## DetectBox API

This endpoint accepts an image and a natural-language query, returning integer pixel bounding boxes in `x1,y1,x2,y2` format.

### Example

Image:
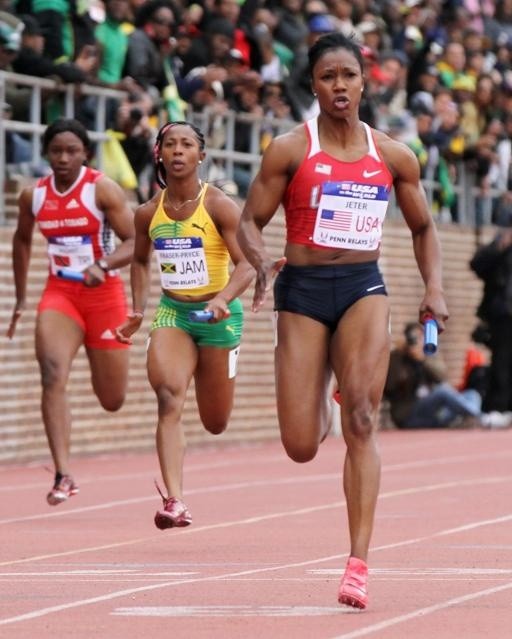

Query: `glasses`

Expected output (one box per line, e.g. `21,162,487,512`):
154,15,174,30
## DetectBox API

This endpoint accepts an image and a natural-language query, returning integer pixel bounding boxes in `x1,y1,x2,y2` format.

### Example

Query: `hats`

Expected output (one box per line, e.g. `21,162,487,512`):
307,14,332,30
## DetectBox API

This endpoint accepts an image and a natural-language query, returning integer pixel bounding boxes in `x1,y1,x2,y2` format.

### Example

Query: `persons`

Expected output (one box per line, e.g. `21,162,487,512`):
234,30,450,609
1,1,512,228
5,117,135,507
382,322,512,429
114,120,258,531
468,191,512,413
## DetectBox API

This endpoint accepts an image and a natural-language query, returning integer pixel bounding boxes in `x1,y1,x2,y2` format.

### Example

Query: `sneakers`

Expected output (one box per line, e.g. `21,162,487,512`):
152,480,194,530
481,409,512,428
336,556,369,610
46,470,80,507
